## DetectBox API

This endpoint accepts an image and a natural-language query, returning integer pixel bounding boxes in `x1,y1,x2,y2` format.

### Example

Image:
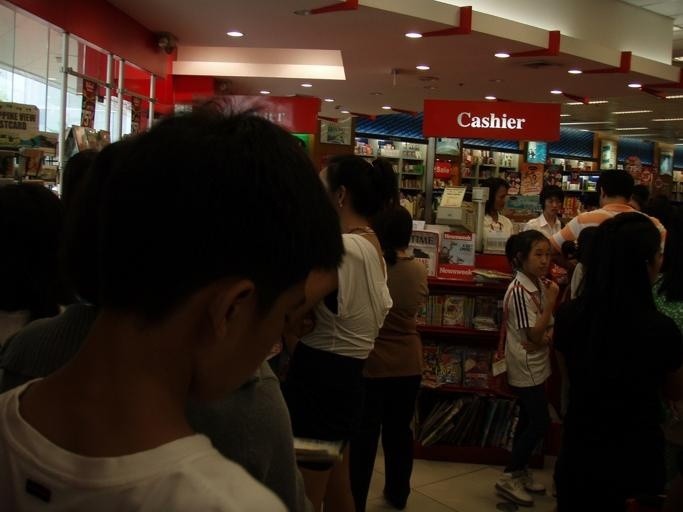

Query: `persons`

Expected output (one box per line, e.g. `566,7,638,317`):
481,176,565,243
0,96,347,512
549,170,683,511
495,230,559,507
271,155,428,511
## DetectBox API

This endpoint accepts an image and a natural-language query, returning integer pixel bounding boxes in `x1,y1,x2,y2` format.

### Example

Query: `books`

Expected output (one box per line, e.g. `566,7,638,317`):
411,227,520,453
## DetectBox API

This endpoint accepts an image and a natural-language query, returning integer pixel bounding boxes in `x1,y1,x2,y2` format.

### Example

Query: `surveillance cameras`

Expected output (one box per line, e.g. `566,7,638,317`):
159,33,175,54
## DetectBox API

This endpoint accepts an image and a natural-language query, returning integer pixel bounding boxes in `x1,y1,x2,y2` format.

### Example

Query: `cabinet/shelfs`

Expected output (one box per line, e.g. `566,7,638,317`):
542,151,600,223
354,135,427,222
404,276,547,470
671,169,682,205
432,135,522,223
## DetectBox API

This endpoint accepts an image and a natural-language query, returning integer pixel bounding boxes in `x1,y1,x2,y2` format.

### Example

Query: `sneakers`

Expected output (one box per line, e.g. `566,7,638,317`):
494,470,546,507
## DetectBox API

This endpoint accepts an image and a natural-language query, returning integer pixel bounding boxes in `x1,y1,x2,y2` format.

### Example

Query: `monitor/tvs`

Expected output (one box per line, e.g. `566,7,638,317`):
436,201,479,233
